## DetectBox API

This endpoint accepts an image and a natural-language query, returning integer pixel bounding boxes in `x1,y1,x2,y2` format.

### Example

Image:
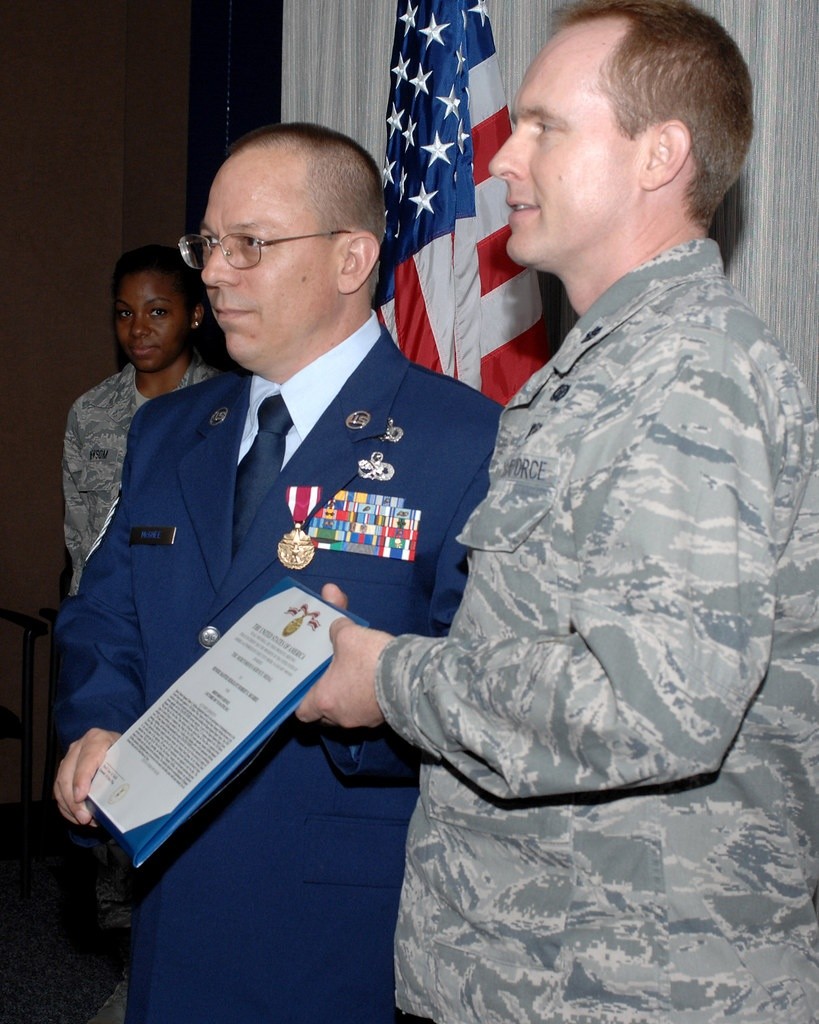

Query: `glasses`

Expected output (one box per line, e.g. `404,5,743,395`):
178,229,354,270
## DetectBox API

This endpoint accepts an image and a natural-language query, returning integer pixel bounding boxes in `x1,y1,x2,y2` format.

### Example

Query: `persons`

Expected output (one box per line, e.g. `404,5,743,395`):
61,245,219,597
291,66,817,1024
53,121,505,1023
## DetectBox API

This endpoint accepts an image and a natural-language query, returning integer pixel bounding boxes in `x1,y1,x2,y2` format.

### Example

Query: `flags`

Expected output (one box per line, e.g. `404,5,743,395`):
376,0,548,409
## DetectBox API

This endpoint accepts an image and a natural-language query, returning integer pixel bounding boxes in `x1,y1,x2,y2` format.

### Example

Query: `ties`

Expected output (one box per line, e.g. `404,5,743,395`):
231,394,293,565
